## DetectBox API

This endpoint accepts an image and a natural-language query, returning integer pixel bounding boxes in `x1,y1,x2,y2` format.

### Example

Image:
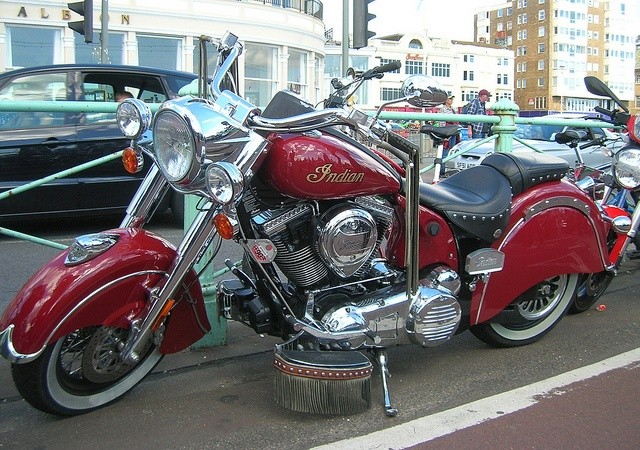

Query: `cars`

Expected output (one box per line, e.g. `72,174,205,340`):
444,113,627,177
384,123,409,139
1,64,210,231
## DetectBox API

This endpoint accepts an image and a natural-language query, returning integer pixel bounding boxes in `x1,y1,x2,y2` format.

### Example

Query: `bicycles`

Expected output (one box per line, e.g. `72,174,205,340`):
554,131,614,195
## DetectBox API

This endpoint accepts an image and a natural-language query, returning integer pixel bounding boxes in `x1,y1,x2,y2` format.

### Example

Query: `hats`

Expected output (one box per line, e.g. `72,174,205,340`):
479,90,492,96
448,94,455,98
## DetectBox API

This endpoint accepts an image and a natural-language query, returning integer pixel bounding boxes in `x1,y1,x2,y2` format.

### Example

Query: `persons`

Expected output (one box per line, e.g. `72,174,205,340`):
467,90,492,138
439,95,458,158
115,91,134,103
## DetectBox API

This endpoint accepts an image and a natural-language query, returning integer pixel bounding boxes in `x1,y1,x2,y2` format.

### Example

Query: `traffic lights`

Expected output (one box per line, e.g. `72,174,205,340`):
354,0,376,49
67,1,93,42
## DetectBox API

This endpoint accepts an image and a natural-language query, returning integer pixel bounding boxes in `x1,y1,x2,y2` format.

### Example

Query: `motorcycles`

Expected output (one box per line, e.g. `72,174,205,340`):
570,77,639,315
0,29,613,417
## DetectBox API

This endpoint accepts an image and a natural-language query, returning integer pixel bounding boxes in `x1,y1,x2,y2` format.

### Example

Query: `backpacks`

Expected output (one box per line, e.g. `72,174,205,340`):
459,99,479,128
